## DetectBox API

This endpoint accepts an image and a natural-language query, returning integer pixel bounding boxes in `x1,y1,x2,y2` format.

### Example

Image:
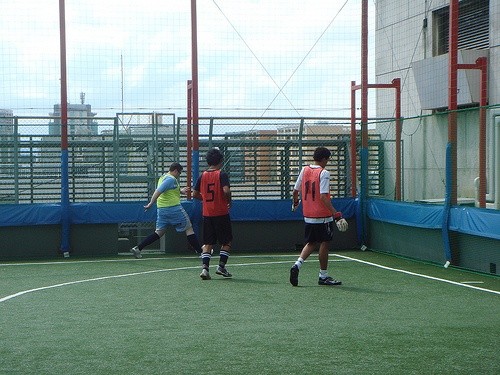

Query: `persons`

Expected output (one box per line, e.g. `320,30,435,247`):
192,149,234,280
289,147,349,287
131,162,214,259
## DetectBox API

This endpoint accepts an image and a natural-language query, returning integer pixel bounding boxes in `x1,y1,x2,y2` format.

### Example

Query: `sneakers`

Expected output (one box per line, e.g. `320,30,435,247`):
318,277,341,285
200,269,211,280
197,249,214,256
290,265,299,287
132,246,142,258
215,266,232,277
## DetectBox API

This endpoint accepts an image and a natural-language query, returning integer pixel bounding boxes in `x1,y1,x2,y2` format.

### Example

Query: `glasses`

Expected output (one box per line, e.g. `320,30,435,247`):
177,169,181,174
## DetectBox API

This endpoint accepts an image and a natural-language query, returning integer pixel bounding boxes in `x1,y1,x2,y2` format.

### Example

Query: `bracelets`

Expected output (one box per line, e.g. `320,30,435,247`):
333,212,342,218
294,199,299,204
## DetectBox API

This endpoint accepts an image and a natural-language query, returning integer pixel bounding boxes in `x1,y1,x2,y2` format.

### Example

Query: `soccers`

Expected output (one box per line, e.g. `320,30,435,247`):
202,244,213,256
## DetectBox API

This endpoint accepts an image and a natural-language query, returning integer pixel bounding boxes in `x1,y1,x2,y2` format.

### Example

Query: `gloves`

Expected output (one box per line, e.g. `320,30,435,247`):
334,212,348,232
292,200,301,212
